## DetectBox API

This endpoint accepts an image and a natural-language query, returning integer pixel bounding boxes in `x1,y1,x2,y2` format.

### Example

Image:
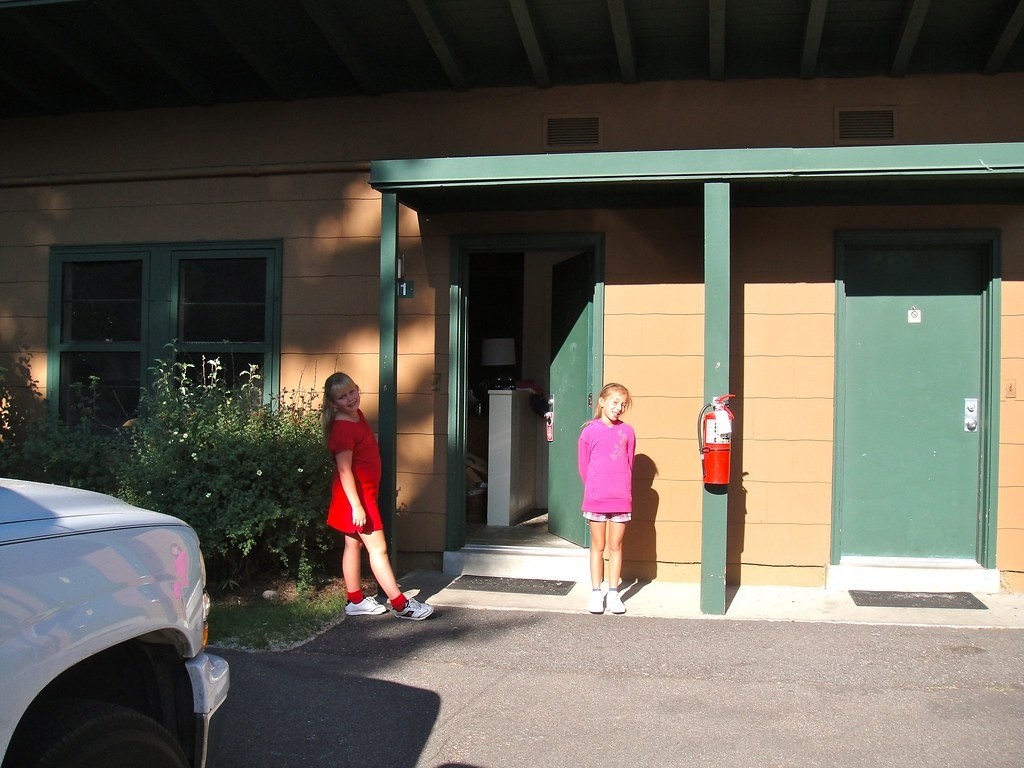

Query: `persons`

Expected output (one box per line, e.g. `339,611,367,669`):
578,382,637,614
319,371,436,621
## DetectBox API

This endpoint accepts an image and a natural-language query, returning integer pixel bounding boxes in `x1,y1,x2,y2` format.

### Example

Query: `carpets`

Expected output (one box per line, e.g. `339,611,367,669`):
846,590,989,606
445,574,578,596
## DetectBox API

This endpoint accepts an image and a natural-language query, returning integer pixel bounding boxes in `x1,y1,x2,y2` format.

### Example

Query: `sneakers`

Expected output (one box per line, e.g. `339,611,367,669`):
389,597,435,620
603,593,626,614
344,595,387,615
588,591,604,613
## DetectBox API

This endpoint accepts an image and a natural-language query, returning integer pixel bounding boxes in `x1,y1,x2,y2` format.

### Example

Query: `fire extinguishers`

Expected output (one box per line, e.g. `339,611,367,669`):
698,393,736,484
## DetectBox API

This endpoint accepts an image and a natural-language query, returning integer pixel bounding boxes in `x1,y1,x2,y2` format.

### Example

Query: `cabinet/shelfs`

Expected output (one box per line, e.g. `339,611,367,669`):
489,390,550,527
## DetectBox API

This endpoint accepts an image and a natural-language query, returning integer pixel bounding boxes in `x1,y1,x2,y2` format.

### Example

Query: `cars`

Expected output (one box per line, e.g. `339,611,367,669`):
0,476,232,767
